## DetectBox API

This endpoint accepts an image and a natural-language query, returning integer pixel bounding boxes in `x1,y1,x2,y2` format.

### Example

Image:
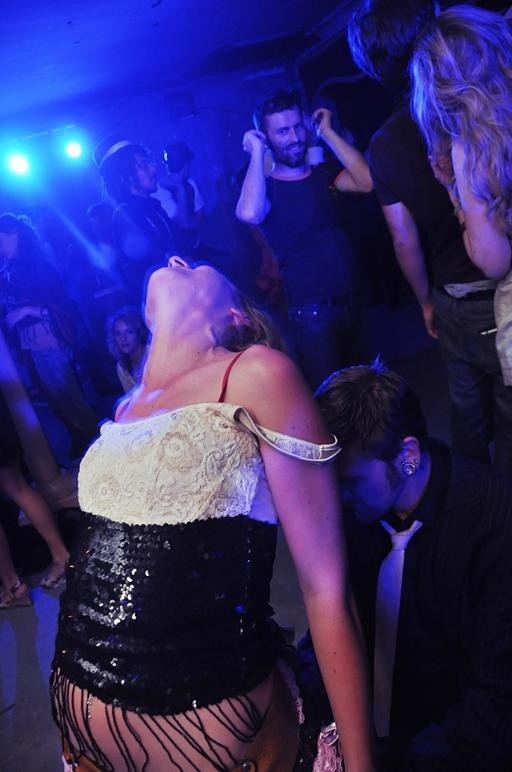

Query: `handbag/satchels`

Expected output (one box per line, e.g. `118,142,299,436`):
54,296,93,351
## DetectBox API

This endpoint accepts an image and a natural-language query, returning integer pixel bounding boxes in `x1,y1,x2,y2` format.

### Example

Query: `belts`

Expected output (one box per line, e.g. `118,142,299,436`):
371,520,423,738
442,287,494,302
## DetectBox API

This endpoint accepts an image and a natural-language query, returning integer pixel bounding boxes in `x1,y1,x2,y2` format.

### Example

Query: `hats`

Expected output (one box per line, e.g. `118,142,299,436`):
160,141,196,164
93,134,142,175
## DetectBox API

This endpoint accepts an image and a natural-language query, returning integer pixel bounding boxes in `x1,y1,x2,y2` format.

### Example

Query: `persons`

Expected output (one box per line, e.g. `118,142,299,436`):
1,2,511,771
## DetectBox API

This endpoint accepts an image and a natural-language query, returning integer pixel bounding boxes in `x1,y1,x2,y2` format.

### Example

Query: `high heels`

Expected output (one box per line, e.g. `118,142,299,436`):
0,577,33,609
38,552,72,588
